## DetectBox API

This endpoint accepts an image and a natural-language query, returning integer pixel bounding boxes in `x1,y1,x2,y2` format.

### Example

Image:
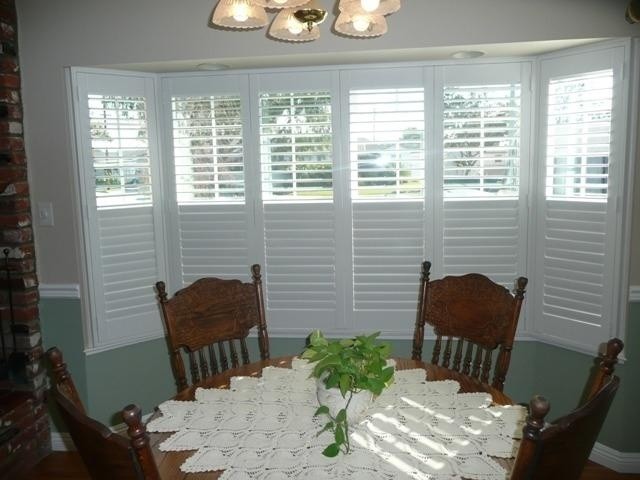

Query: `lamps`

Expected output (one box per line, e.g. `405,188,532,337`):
206,0,403,46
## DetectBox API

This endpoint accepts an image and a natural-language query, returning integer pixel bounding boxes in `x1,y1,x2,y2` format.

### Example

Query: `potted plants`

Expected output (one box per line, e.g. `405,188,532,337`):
297,325,397,460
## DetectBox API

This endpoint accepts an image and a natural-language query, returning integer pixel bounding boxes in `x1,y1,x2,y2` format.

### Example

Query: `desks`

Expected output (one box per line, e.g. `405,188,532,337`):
135,350,529,479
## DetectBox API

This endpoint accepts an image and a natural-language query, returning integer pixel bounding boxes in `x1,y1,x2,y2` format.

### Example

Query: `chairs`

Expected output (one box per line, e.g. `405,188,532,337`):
42,343,166,480
409,258,529,396
155,262,273,393
505,337,625,480
